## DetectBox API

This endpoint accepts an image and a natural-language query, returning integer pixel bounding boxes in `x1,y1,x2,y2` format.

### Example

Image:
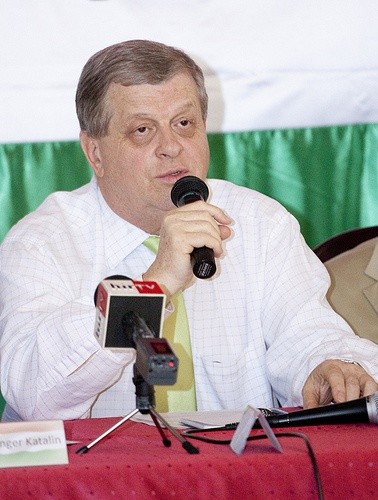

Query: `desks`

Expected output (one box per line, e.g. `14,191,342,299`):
0,416,378,500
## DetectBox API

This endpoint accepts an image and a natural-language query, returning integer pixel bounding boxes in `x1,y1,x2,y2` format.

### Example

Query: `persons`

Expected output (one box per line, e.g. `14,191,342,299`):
0,40,378,420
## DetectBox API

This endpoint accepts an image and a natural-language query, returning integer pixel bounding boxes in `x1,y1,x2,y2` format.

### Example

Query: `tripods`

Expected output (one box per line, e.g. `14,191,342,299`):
75,365,200,457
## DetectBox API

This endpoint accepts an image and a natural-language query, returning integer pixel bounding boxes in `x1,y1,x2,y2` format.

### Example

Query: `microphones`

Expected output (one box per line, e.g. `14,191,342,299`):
171,175,217,279
94,275,179,387
225,393,378,431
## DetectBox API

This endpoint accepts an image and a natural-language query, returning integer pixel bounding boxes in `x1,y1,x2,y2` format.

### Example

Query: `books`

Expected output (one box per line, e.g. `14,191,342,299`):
179,408,282,430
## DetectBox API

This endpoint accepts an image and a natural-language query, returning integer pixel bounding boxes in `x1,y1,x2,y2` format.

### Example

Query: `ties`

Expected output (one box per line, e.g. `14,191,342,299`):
144,237,196,413
